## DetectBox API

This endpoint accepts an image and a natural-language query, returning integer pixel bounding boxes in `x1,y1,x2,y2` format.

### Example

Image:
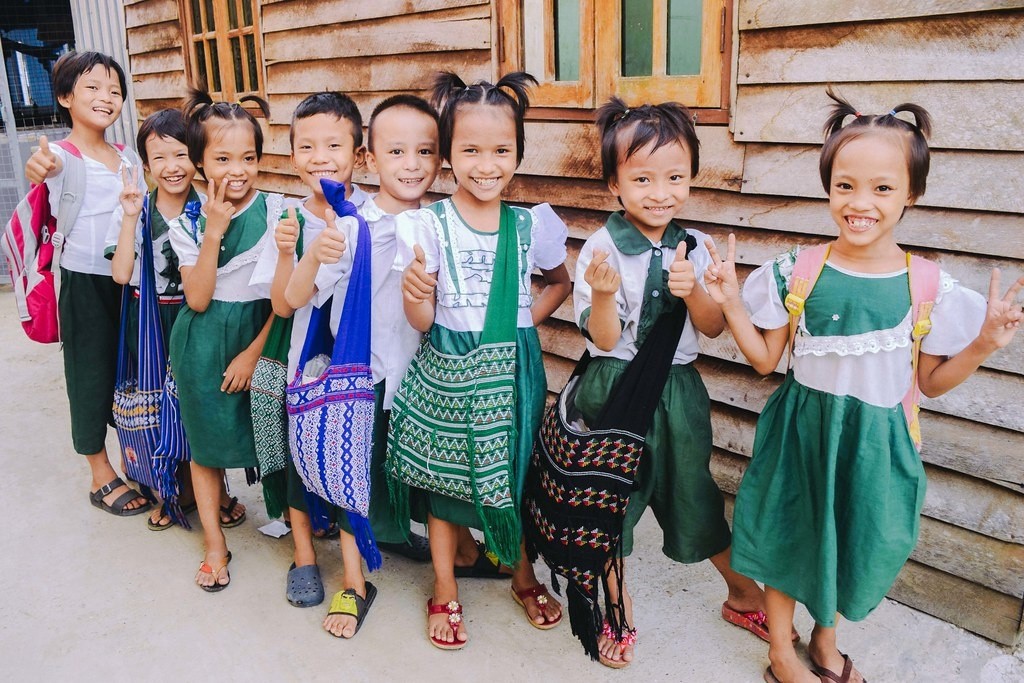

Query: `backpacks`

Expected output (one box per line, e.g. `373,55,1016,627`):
111,380,165,491
785,244,941,454
0,139,140,345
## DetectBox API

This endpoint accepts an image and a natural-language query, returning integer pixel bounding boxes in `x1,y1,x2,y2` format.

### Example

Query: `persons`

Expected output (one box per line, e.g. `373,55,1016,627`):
573,94,801,668
26,51,153,517
104,70,572,652
704,80,1023,683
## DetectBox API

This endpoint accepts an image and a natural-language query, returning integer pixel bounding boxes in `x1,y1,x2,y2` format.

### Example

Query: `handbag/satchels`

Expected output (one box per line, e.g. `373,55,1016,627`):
383,329,523,573
285,362,383,574
249,355,284,482
520,375,646,663
152,357,193,532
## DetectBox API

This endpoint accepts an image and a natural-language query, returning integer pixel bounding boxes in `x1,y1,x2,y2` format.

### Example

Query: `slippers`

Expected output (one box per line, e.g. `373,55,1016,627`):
199,550,232,592
452,539,515,580
763,665,830,683
596,607,638,669
325,581,378,639
722,599,801,647
286,561,325,608
425,597,468,650
219,496,246,528
510,582,563,630
374,530,434,563
147,500,197,531
89,473,151,516
809,646,867,683
285,515,340,539
